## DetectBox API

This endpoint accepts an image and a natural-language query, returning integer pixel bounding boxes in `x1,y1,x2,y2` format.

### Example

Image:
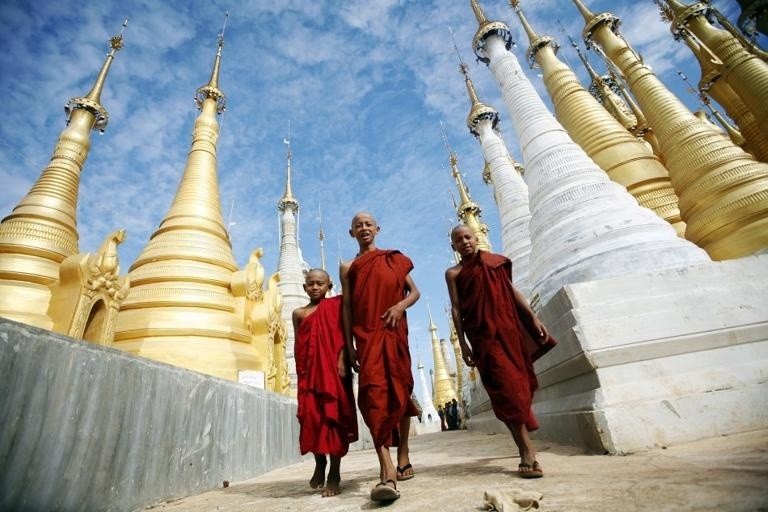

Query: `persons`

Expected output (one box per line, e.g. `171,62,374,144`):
444,225,556,478
437,399,458,432
340,213,421,501
292,269,348,498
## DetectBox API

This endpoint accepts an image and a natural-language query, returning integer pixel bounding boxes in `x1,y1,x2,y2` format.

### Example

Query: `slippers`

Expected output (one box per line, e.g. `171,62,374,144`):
397,463,415,480
518,460,543,477
371,479,400,501
